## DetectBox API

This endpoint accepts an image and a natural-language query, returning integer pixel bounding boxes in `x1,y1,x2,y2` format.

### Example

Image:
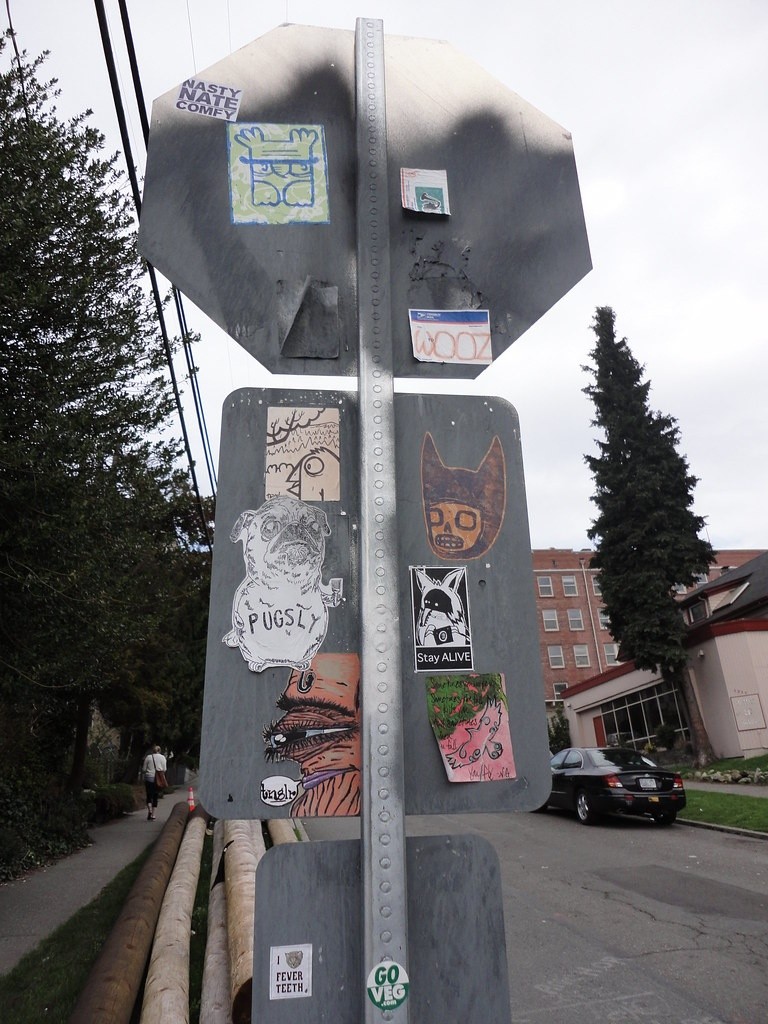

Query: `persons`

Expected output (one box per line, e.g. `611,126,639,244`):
143,746,166,820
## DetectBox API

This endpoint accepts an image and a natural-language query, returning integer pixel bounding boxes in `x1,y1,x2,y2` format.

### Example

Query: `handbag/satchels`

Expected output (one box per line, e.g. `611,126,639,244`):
154,770,168,790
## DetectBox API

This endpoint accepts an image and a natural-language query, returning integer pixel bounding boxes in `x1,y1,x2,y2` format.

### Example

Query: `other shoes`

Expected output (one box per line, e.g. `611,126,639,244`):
147,811,153,820
151,816,155,821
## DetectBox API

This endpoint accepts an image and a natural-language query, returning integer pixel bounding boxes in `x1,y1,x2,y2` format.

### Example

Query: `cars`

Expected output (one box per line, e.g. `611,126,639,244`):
548,747,687,827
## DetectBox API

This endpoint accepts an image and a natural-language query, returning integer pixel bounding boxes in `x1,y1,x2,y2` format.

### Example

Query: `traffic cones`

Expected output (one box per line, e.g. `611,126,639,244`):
187,786,195,811
